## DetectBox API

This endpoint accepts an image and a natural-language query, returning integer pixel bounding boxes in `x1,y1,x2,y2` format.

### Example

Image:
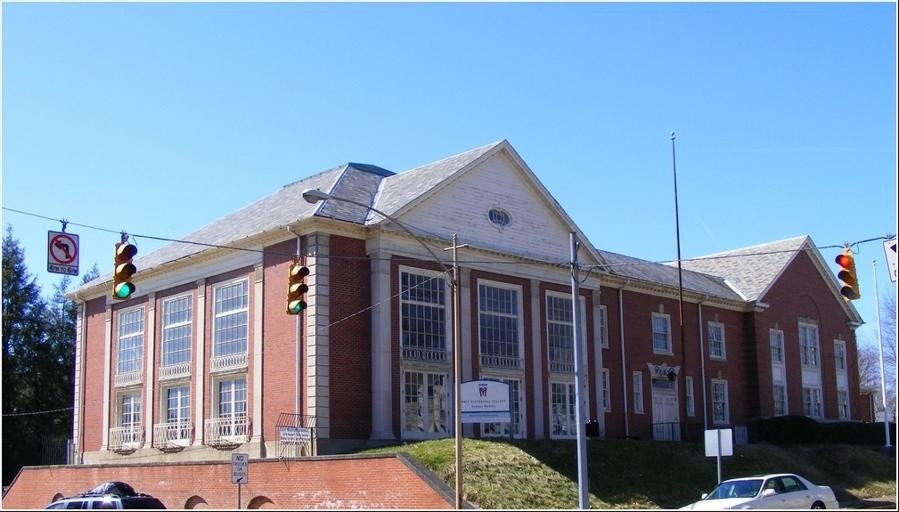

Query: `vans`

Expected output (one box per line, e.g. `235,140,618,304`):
44,493,168,509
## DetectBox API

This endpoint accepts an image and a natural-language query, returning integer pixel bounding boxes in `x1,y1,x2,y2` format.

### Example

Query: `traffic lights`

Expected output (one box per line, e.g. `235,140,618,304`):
835,247,862,302
112,243,138,301
285,264,310,316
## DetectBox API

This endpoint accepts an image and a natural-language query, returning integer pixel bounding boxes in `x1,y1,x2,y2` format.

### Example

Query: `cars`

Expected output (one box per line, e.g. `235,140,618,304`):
676,472,840,511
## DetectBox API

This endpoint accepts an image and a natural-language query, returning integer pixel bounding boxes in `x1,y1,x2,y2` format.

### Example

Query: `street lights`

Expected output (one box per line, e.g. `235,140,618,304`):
301,187,469,509
